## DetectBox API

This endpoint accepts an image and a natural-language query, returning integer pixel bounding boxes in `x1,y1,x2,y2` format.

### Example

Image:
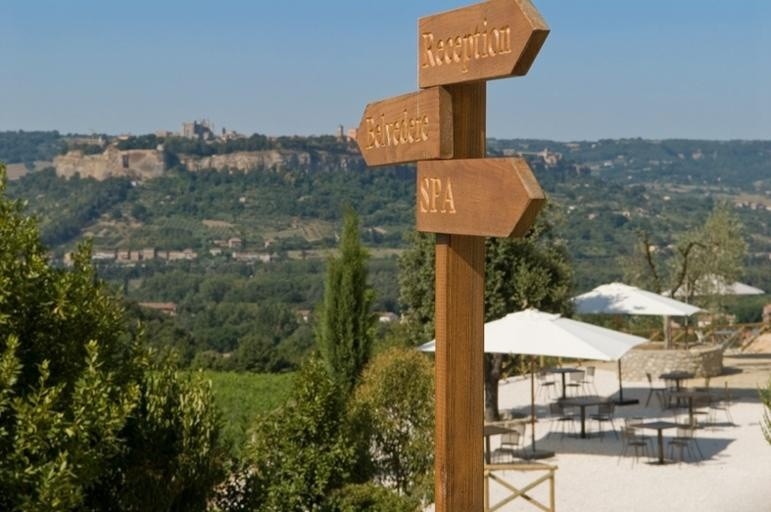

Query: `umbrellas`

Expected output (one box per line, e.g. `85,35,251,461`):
416,304,650,451
557,278,708,401
655,269,765,340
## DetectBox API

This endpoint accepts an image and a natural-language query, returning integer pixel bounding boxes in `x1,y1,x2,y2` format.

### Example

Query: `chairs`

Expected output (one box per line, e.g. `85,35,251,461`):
493,366,733,468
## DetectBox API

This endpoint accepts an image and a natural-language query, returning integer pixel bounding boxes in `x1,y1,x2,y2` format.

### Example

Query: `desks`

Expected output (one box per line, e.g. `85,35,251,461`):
484,425,516,464
